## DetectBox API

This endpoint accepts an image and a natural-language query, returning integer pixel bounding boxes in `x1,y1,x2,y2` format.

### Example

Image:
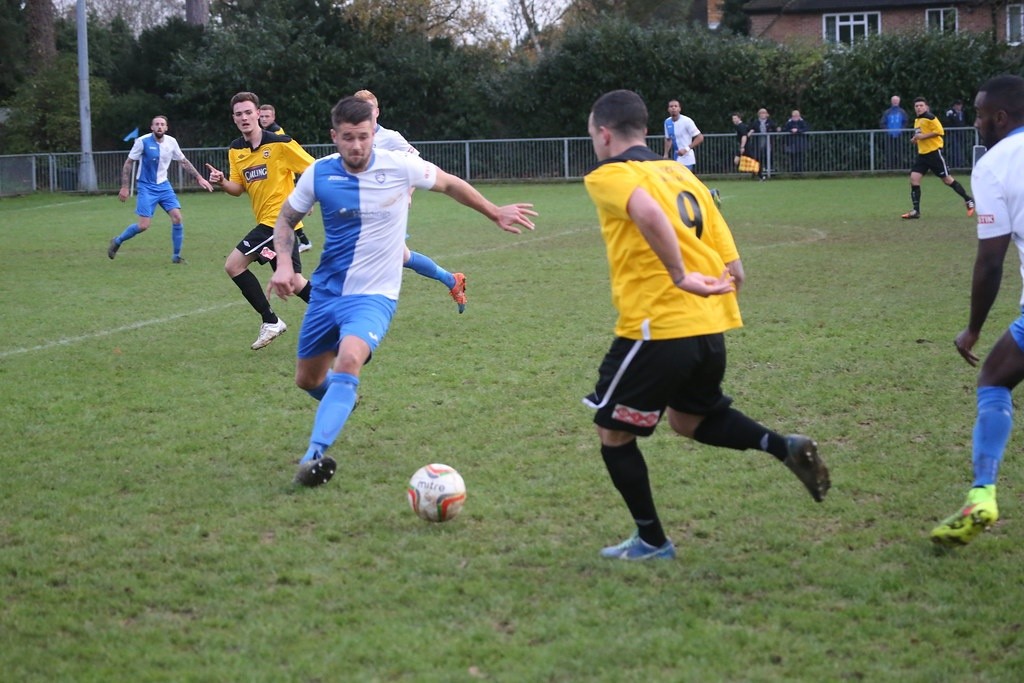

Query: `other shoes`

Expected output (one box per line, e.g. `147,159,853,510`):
712,189,721,209
901,209,920,218
173,256,188,264
966,197,975,217
108,235,120,260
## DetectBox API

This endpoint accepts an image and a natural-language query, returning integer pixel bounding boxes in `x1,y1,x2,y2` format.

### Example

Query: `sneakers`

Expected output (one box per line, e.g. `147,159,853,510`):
930,486,998,549
780,434,831,504
601,530,676,562
294,456,336,488
449,273,467,314
250,317,286,351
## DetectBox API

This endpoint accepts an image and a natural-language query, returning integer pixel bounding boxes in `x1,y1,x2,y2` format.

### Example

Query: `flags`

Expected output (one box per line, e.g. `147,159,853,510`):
124,128,138,142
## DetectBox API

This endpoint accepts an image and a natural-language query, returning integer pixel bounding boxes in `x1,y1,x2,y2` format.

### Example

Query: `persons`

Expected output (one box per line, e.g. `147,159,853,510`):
108,115,214,265
206,91,316,350
663,95,973,218
353,89,466,313
266,97,539,493
931,73,1024,547
583,89,832,560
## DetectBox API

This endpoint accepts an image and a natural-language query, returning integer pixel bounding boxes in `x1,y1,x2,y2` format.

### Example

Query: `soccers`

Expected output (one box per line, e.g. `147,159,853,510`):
407,462,468,523
